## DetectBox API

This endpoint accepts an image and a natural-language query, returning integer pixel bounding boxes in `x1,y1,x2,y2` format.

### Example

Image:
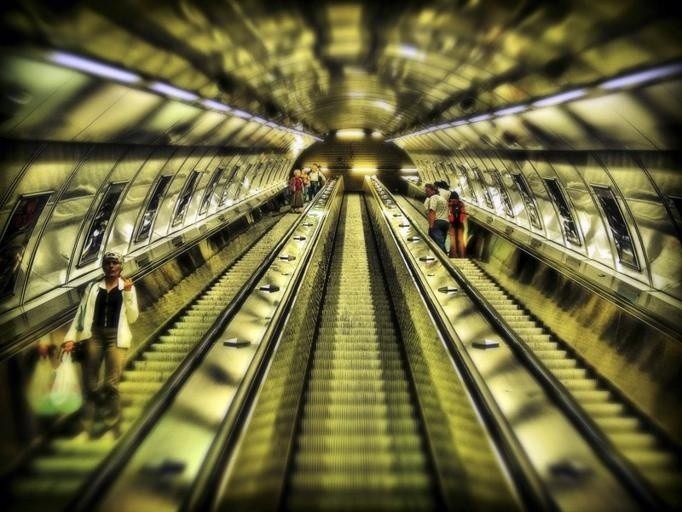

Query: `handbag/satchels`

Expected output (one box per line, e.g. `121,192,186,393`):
290,184,296,191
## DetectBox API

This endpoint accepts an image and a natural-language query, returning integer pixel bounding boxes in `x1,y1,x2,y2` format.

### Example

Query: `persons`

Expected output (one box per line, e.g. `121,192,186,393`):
289,163,326,215
59,252,141,435
423,179,469,260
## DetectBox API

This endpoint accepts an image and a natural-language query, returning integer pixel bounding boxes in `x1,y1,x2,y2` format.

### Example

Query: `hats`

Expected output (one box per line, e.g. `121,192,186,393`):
101,252,124,271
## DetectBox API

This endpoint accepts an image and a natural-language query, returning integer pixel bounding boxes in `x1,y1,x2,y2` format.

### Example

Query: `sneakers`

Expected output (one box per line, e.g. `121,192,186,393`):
290,209,298,213
91,406,122,436
